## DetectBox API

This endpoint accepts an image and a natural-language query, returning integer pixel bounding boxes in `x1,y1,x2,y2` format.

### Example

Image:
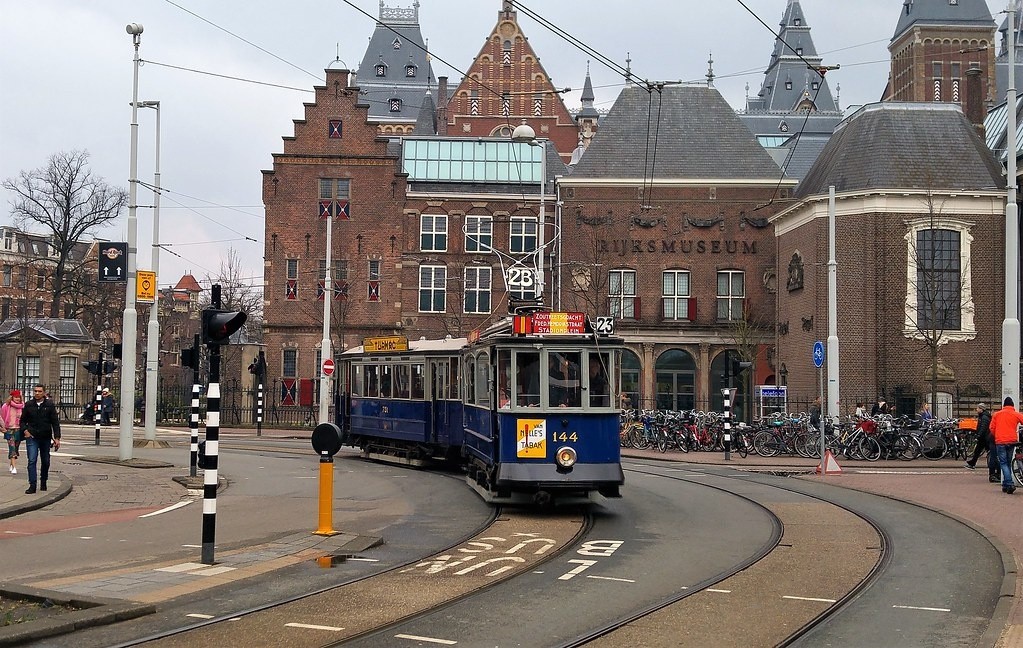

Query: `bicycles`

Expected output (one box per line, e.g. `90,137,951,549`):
1012,428,1023,486
620,400,991,461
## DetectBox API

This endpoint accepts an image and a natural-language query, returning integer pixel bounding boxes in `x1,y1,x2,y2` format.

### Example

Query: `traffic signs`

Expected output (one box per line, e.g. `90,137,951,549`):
97,242,127,283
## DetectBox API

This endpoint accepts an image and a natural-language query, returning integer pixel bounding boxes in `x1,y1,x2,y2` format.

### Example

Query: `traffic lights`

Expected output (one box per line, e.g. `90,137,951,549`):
82,361,97,375
200,308,247,347
181,347,195,369
247,357,260,374
104,361,117,374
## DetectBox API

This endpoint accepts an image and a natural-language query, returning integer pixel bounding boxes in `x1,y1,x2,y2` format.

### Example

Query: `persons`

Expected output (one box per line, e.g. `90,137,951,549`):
855,396,896,418
102,387,113,425
964,397,1023,494
621,392,634,412
527,354,608,408
18,384,61,493
810,396,822,429
0,388,25,474
919,402,932,420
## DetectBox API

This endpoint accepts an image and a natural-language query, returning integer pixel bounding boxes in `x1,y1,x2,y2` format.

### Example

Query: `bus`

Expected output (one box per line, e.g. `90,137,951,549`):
334,224,626,509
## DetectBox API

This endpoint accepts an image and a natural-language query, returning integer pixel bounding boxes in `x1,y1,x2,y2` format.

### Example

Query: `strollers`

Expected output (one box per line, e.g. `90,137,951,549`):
78,398,97,425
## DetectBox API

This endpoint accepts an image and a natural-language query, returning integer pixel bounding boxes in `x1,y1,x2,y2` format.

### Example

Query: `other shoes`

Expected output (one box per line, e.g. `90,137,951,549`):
101,422,113,426
1002,485,1017,494
965,460,976,469
25,486,47,494
988,473,1001,483
8,464,18,474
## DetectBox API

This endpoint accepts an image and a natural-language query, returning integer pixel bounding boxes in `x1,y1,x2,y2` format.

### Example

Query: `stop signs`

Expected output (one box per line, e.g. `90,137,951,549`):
323,359,334,375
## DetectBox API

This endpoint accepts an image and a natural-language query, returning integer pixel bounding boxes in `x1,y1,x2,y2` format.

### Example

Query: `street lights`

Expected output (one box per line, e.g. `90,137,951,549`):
117,20,145,461
721,348,753,458
127,101,162,442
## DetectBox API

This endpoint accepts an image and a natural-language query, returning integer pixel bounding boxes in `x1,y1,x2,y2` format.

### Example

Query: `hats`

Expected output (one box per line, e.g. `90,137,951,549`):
102,387,110,393
978,402,987,410
1003,397,1015,407
9,390,22,397
877,400,886,409
